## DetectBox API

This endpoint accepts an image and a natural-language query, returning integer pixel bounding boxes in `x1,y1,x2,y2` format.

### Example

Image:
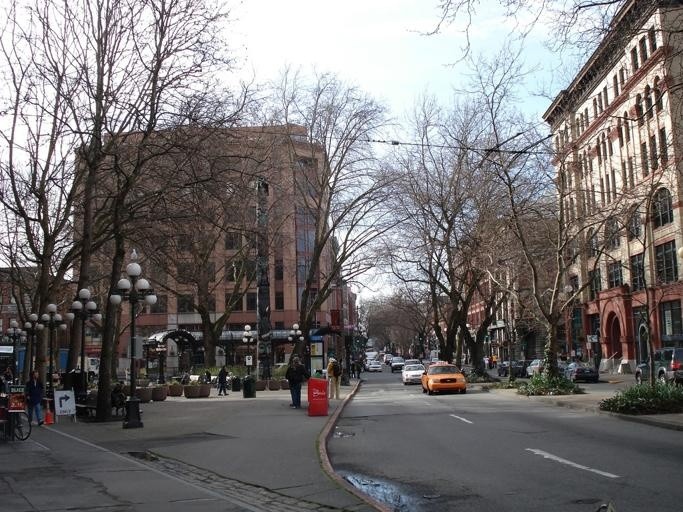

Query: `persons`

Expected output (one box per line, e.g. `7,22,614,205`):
325,356,341,400
492,354,498,368
285,357,308,408
216,365,229,397
24,369,44,427
350,359,364,379
487,355,493,369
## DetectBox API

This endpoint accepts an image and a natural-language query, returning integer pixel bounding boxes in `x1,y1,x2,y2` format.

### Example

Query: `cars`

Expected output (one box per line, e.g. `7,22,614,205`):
365,348,467,395
498,359,599,383
275,363,288,369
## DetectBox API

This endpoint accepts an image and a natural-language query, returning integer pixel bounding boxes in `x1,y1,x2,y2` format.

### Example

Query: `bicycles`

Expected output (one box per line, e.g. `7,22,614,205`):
7,412,32,442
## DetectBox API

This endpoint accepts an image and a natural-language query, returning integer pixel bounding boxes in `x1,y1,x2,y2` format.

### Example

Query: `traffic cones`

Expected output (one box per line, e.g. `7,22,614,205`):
44,400,56,426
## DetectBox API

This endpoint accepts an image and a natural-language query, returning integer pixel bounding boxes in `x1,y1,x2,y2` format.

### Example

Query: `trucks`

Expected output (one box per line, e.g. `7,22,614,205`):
76,355,100,380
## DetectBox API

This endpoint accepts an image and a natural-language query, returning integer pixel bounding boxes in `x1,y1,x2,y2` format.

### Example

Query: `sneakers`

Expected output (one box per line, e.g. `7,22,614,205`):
37,420,45,427
218,394,229,396
289,404,300,409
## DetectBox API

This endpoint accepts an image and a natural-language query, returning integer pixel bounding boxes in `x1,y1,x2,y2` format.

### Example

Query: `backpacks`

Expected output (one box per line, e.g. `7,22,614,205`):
331,360,344,377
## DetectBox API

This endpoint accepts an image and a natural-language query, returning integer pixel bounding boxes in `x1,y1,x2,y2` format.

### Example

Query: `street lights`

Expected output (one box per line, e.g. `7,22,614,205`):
24,312,44,373
564,285,578,361
65,286,102,404
241,324,255,374
5,320,27,385
464,322,470,364
110,259,158,431
36,303,66,399
287,323,305,355
153,344,166,384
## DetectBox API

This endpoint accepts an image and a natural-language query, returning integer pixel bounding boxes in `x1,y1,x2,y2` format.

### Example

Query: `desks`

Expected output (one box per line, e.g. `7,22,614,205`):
171,376,182,383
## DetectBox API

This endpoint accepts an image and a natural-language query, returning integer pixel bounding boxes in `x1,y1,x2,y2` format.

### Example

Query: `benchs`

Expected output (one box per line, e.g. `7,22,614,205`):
76,403,126,422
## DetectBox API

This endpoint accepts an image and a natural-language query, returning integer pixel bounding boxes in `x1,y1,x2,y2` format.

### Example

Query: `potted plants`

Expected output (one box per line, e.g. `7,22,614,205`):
136,379,152,403
268,376,280,390
197,379,211,397
183,380,200,397
153,383,167,402
231,376,240,391
167,378,183,395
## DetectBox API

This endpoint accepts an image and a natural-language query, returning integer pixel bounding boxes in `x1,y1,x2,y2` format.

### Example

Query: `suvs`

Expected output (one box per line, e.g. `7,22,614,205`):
634,347,683,386
